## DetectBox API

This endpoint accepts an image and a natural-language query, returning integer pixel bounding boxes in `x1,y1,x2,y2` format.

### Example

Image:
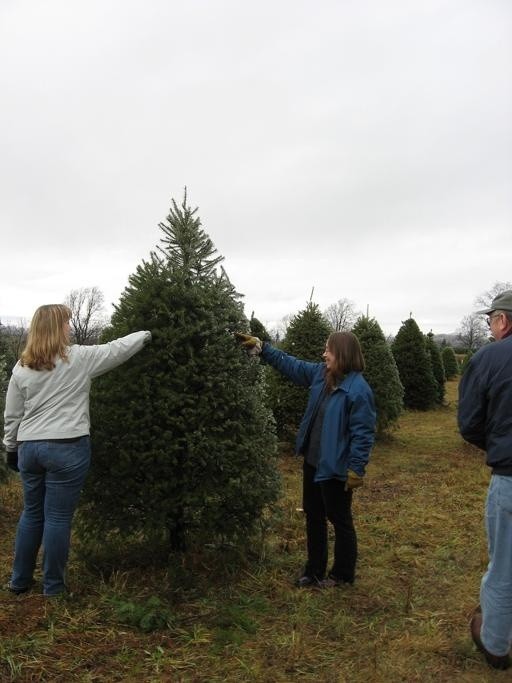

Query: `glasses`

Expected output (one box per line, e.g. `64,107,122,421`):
486,315,499,327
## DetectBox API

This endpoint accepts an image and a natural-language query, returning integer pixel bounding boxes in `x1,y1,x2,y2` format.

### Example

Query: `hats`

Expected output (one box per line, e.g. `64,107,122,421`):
476,290,512,314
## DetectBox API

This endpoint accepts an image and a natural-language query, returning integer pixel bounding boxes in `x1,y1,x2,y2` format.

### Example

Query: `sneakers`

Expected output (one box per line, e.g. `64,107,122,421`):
472,615,510,669
9,578,36,593
296,575,314,586
318,578,338,588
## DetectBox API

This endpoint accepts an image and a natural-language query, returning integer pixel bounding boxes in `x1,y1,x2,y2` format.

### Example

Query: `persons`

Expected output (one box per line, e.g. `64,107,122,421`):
236,330,377,593
2,303,153,599
455,287,511,670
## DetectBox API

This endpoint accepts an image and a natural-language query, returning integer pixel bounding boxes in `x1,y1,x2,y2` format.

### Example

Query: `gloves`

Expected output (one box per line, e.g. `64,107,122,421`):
6,451,19,472
344,469,362,492
234,331,264,352
